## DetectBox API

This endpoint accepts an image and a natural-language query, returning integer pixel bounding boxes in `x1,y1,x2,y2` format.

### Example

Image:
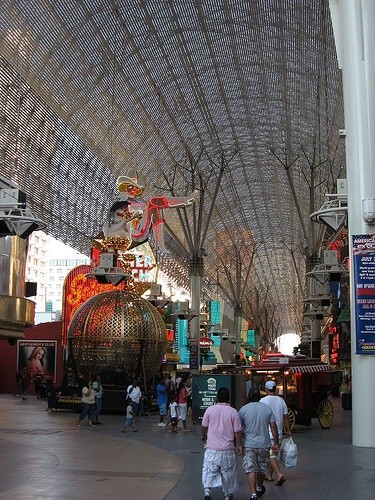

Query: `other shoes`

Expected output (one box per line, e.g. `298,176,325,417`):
204,489,212,500
167,426,182,434
182,428,193,433
90,421,101,426
222,493,233,500
77,420,81,427
122,426,139,433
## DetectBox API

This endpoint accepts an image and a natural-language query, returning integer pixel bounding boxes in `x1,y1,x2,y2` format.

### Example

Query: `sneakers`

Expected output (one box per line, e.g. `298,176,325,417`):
250,494,259,500
257,485,266,497
157,422,166,427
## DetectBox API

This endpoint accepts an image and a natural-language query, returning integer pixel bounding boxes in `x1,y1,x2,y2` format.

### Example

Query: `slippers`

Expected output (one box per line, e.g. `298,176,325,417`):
264,475,275,481
275,479,286,486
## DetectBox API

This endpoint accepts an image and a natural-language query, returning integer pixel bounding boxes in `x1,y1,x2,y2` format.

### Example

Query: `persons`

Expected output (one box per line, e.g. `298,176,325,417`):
34,370,53,400
176,380,192,432
186,376,192,415
126,379,147,427
157,379,167,427
121,400,138,432
10,367,29,399
76,379,97,427
25,347,46,376
235,388,280,500
91,374,103,425
167,391,182,432
45,376,57,412
200,387,242,500
259,380,292,486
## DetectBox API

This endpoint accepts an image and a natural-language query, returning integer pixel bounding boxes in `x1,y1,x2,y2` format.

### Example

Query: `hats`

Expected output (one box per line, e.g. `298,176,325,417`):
265,381,276,389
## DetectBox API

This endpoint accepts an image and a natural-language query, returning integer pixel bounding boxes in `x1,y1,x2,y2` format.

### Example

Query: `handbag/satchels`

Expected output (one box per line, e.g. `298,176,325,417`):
279,436,297,467
